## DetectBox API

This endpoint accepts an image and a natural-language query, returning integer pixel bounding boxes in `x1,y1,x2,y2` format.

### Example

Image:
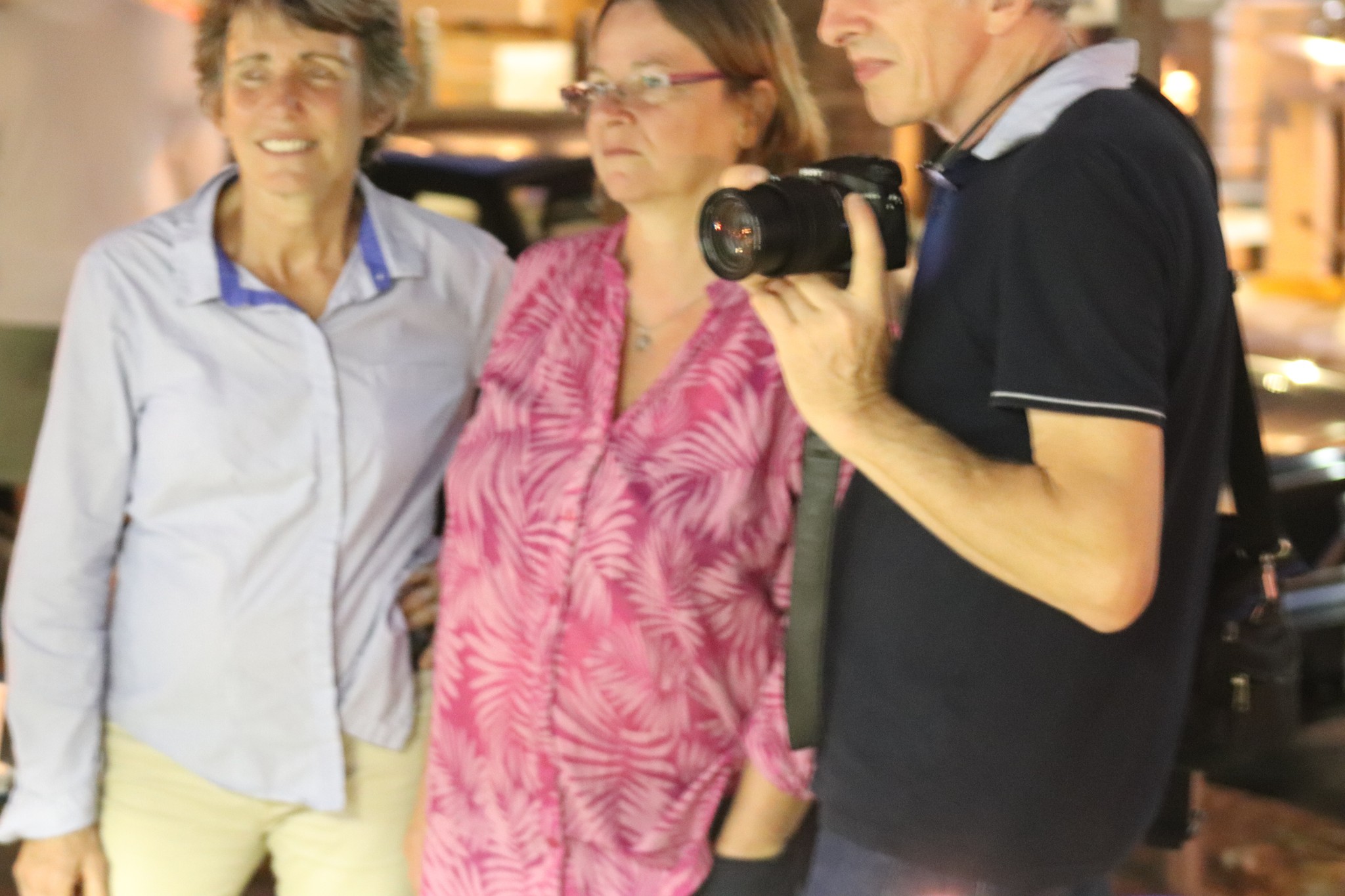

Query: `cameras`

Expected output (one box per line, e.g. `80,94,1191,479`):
697,154,909,284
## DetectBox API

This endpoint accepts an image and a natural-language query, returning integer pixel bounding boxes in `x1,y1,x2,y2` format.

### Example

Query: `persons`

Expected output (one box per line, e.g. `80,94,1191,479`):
0,0,521,896
410,0,901,896
720,0,1233,896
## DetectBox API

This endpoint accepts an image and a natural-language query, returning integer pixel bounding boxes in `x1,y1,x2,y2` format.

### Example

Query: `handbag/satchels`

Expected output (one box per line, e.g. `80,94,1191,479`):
1200,592,1306,791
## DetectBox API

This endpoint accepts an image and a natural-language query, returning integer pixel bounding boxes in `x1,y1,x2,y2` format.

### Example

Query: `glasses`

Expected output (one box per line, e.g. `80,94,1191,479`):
558,62,734,121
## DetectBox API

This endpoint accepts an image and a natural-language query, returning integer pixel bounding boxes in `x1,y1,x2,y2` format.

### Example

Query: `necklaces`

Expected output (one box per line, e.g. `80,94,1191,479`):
626,294,700,348
